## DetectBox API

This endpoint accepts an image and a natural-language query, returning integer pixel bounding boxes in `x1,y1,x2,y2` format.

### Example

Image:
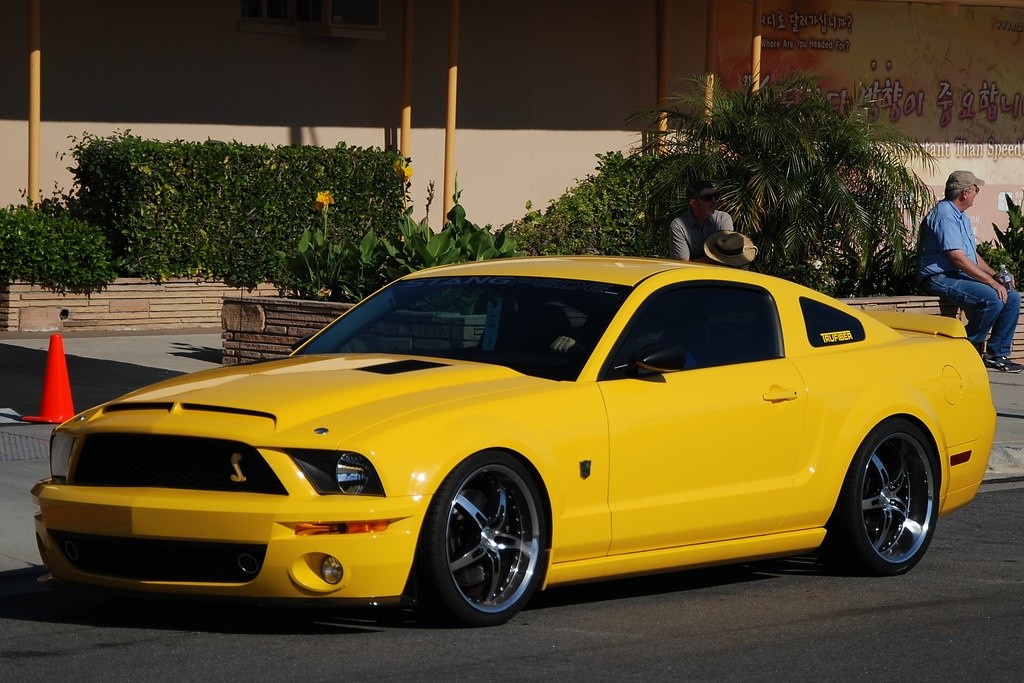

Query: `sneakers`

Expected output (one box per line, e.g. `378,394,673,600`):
982,355,1024,372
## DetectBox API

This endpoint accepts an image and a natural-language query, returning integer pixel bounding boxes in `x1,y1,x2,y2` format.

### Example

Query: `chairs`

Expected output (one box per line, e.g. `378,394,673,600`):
502,305,573,349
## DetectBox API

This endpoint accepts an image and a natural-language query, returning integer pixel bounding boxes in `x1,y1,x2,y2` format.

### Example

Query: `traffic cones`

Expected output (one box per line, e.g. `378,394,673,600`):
21,332,80,425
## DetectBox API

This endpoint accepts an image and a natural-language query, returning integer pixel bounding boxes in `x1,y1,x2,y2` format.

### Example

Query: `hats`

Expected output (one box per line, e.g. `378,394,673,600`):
704,230,757,267
946,171,985,191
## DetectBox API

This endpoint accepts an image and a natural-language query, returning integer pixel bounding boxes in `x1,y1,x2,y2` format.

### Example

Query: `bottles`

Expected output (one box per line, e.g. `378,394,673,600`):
1000,265,1013,293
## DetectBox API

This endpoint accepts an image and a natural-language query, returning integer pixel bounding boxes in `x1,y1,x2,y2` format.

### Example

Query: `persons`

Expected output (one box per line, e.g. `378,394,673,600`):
914,171,1024,374
669,179,735,264
549,303,696,384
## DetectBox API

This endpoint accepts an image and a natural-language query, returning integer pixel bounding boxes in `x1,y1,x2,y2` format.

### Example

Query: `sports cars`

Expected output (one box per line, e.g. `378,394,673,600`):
28,257,1000,628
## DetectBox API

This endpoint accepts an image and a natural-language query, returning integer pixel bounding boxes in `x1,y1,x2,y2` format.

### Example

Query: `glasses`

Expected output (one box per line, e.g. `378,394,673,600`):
966,187,979,194
690,192,720,202
